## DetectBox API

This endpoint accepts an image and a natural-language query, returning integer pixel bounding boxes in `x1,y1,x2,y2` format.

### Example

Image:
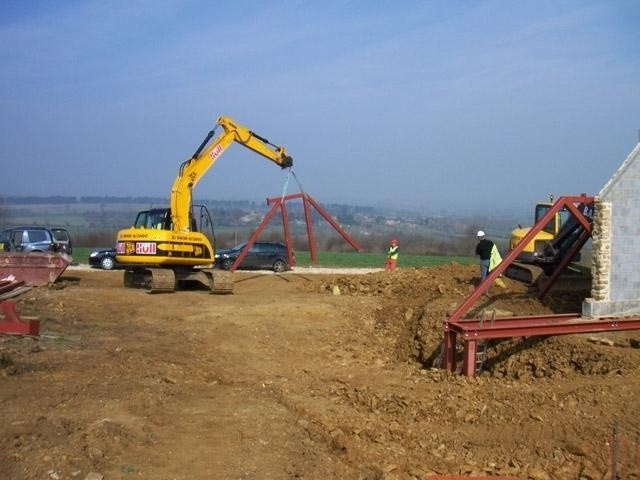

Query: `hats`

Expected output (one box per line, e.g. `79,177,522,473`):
389,240,398,244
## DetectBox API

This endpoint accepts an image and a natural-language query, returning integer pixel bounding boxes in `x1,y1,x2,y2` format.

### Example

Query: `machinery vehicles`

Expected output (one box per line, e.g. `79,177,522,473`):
504,195,595,295
111,114,292,295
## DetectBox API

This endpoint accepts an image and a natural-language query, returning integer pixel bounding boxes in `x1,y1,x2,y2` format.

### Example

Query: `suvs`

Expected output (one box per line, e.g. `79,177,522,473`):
0,226,72,256
214,241,296,273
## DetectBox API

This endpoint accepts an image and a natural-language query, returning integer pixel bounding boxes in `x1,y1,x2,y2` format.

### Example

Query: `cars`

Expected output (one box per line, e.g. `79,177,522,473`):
89,246,145,270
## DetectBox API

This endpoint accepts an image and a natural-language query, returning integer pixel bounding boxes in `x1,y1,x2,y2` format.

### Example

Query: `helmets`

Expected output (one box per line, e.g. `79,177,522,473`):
477,231,485,237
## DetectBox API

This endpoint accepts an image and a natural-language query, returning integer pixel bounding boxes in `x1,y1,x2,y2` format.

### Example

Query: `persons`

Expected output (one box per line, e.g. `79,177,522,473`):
385,239,400,272
475,230,502,294
